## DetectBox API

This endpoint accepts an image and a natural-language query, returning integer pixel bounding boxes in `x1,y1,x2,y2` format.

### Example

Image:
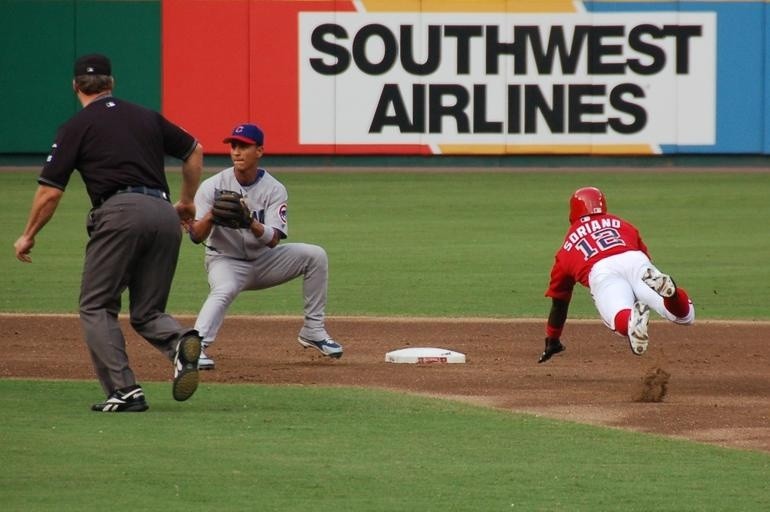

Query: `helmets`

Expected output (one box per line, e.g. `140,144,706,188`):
568,185,607,222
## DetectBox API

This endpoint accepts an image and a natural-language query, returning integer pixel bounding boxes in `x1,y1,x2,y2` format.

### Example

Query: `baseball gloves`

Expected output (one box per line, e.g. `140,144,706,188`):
209,190,255,229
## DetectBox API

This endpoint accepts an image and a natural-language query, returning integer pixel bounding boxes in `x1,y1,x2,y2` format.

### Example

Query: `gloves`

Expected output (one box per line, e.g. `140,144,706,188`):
537,336,566,364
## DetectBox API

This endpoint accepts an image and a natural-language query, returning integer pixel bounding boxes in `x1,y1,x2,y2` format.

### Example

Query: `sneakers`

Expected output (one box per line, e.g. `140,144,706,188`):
91,384,149,412
172,335,201,402
640,264,676,298
628,300,650,356
196,346,215,370
296,326,343,359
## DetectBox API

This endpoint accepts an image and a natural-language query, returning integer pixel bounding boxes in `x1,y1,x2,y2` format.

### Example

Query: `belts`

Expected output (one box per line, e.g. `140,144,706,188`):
94,186,170,207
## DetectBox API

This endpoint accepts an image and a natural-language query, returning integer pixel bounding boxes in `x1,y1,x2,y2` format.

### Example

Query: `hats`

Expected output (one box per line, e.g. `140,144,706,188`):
74,54,111,76
222,123,264,146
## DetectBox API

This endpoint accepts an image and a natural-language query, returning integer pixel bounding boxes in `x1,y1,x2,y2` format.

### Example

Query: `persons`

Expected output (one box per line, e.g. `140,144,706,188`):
539,187,695,363
14,50,204,412
182,123,344,370
